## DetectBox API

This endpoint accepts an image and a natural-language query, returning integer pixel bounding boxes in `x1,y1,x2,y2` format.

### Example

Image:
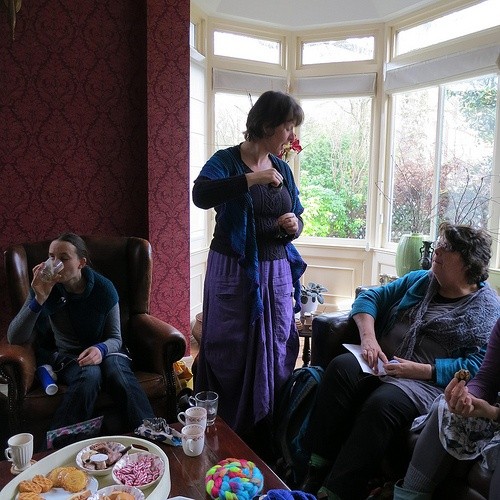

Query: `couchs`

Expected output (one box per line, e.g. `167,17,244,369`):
0,235,187,453
284,284,500,500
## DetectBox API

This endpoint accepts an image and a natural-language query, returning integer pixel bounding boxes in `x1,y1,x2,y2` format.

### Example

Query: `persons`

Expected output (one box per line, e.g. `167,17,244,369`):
277,222,500,500
393,319,500,500
8,233,154,449
193,91,307,433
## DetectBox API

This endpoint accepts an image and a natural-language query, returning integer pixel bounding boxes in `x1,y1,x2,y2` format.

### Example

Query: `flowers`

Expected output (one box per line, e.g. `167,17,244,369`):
277,133,302,162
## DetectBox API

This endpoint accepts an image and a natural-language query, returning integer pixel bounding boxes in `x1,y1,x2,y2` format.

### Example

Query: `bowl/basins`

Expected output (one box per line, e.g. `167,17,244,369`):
87,484,144,500
76,441,126,476
112,452,164,489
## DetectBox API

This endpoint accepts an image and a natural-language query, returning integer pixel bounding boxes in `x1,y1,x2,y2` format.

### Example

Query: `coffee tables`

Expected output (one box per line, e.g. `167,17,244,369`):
0,414,290,500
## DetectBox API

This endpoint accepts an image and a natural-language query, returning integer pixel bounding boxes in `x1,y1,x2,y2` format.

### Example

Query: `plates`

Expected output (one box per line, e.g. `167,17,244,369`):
15,474,98,500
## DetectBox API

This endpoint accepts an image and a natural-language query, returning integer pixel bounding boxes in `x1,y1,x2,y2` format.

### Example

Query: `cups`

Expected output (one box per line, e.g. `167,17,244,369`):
5,433,34,471
40,256,65,284
181,424,205,456
188,391,219,426
177,407,207,431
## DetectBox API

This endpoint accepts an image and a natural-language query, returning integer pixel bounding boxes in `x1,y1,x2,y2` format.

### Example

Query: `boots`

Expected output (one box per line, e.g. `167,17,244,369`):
393,478,434,500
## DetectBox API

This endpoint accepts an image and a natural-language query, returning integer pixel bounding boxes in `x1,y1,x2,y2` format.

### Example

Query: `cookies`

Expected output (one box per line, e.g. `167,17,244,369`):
15,467,135,500
455,370,471,385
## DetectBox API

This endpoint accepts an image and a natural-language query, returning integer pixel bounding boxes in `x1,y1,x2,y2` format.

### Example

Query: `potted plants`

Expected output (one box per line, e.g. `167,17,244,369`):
299,282,328,316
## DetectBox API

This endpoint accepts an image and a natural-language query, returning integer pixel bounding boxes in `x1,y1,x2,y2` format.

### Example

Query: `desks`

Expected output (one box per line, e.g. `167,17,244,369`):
295,310,328,368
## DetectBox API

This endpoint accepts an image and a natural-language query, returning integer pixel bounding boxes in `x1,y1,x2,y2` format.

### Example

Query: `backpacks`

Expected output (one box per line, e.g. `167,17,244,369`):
275,365,325,457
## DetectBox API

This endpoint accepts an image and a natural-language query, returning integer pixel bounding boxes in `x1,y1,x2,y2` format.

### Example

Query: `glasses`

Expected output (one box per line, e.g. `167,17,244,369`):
433,240,454,252
